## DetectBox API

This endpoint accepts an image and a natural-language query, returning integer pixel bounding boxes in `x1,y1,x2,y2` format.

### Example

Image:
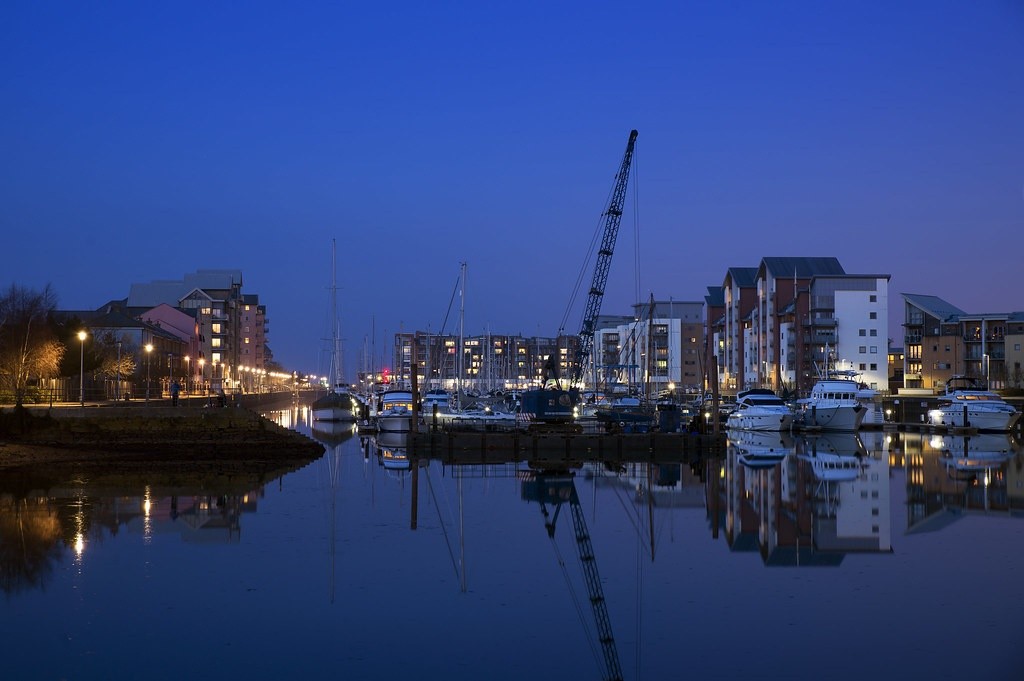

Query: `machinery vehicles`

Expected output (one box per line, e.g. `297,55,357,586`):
521,468,625,681
520,128,640,431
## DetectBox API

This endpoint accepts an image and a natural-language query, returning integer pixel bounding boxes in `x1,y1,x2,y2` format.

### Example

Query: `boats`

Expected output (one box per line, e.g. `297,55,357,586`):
797,343,869,433
938,433,1018,490
726,389,795,431
728,431,796,469
377,433,412,491
376,380,426,432
797,432,869,517
312,320,356,420
927,375,1023,433
312,421,355,488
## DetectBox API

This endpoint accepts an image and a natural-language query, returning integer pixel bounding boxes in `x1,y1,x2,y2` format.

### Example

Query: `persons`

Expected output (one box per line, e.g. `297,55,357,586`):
217,389,226,406
171,381,179,406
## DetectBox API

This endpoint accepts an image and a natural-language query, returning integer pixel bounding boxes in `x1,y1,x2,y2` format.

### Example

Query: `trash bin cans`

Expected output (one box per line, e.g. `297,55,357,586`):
693,416,705,434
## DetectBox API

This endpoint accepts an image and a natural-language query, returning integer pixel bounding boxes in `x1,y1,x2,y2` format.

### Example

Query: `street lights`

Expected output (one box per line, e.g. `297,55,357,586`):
238,365,292,394
144,344,154,401
983,354,989,391
199,359,205,396
77,330,87,402
184,356,190,396
